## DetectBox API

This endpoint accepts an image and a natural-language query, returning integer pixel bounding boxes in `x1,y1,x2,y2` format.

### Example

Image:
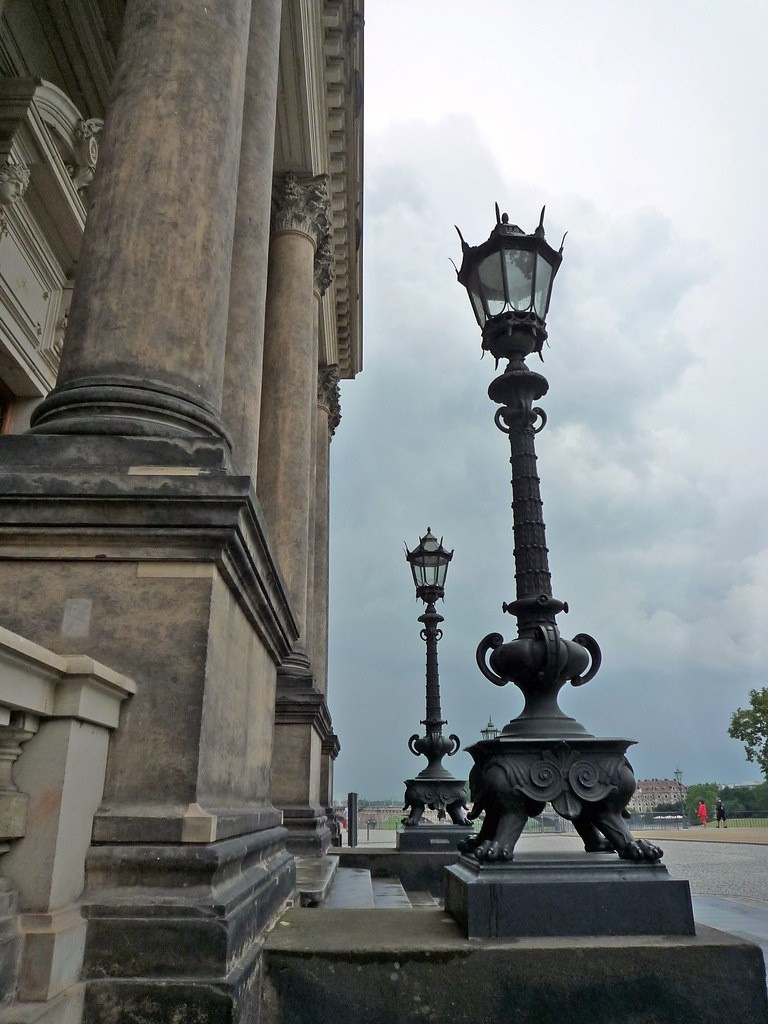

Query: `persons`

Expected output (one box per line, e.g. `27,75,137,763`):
0,162,30,240
344,806,348,832
716,797,727,828
698,801,708,828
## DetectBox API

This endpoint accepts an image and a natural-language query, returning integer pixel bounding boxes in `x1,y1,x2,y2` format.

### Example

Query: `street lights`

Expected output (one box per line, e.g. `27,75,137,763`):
444,202,664,867
400,526,472,828
673,767,688,829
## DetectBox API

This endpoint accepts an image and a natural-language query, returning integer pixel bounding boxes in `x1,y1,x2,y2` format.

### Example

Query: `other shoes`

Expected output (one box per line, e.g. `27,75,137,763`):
724,825,728,828
715,826,720,829
